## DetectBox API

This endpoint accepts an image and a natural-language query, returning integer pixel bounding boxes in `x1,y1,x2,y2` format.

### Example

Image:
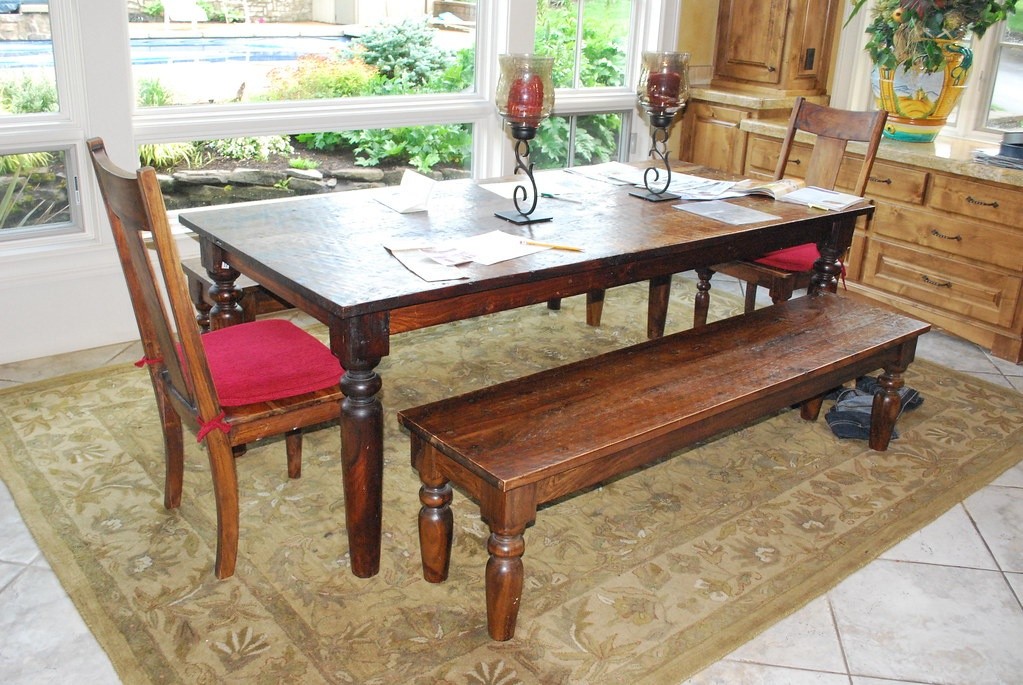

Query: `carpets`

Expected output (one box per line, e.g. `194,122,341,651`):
0,275,1023,685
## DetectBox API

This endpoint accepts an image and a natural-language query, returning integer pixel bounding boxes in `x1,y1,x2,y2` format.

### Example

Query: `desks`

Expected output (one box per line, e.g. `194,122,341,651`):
182,155,878,579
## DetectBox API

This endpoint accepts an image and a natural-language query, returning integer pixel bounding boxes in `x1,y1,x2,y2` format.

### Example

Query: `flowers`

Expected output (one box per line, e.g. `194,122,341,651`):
842,0,1020,76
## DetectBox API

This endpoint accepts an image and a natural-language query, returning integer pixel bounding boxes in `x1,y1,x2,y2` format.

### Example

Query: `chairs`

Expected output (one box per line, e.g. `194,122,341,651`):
84,134,383,583
693,96,891,329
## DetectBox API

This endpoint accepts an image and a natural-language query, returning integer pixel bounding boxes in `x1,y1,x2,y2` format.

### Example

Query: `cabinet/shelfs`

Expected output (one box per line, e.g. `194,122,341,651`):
683,0,1023,365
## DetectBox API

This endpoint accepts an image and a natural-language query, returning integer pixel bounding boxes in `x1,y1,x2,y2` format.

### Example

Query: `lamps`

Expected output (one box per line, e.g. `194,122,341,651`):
492,53,556,225
629,48,693,203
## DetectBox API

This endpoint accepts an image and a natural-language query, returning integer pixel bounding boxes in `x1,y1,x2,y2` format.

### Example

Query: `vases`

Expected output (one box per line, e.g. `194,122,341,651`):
869,28,974,143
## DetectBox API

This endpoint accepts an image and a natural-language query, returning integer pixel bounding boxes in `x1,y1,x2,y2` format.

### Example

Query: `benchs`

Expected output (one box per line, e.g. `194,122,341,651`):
393,290,933,641
183,258,295,327
